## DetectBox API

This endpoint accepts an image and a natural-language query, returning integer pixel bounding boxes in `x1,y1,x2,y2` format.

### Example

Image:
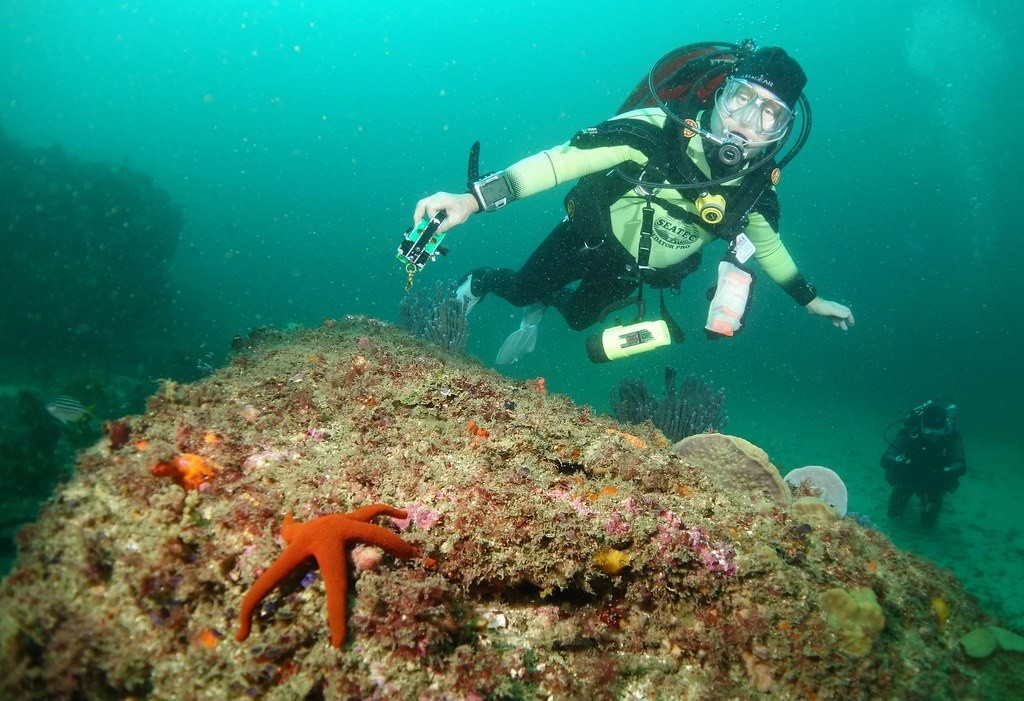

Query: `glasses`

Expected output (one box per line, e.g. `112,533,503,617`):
723,78,791,136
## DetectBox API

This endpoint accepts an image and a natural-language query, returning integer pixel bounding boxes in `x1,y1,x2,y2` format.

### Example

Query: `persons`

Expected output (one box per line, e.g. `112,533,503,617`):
412,36,853,363
880,398,968,529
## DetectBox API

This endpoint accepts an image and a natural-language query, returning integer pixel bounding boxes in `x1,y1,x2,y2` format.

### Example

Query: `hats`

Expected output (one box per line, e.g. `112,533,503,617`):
733,45,807,107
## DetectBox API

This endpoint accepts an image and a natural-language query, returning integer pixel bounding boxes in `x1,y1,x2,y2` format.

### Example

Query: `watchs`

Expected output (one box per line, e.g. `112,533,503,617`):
465,139,517,214
